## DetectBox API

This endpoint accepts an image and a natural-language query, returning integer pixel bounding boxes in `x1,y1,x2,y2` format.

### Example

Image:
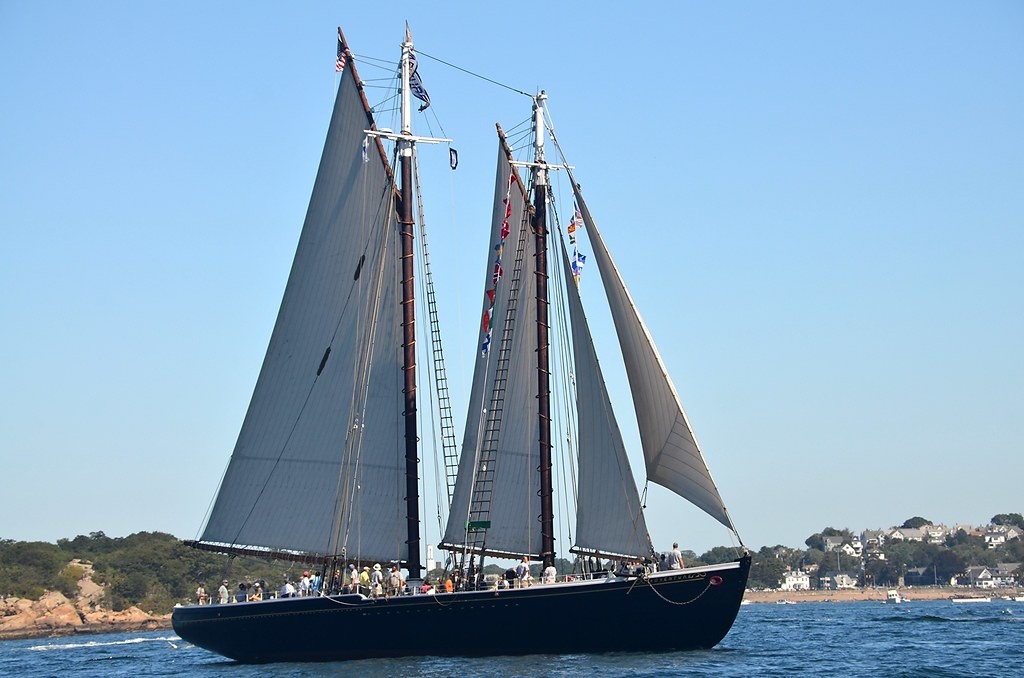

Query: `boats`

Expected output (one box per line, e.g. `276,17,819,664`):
949,596,991,603
776,598,796,605
1006,590,1024,602
880,585,910,605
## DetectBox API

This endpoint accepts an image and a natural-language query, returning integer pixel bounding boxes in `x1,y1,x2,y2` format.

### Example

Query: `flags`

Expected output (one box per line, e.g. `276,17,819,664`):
568,197,586,291
336,29,346,72
361,136,370,164
482,173,516,359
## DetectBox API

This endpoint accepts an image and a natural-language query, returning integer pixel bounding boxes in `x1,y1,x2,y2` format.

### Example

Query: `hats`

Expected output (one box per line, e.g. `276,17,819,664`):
253,583,259,587
284,579,290,583
221,579,229,583
660,554,666,558
372,563,381,571
363,566,370,571
303,571,309,576
349,563,355,570
199,581,205,585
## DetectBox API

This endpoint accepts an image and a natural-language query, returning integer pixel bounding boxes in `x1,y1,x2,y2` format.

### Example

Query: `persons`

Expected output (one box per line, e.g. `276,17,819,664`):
622,555,654,575
582,563,618,580
516,556,533,588
476,572,510,590
217,579,230,604
196,582,213,605
543,561,558,584
348,564,403,598
658,553,668,571
280,569,341,598
669,542,684,570
405,569,467,595
236,580,270,603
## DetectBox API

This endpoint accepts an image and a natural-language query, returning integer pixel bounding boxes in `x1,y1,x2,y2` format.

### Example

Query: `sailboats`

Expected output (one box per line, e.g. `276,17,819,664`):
172,17,752,662
